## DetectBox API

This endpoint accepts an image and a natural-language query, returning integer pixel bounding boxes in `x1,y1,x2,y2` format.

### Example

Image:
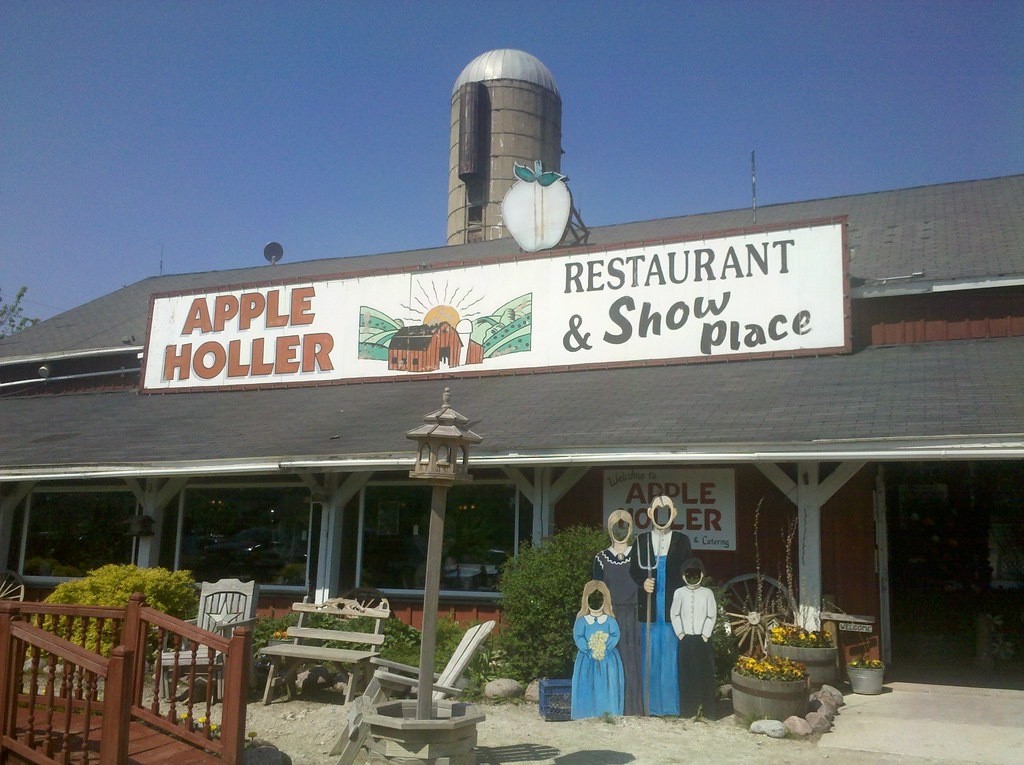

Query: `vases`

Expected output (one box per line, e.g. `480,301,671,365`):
766,642,836,687
731,669,810,725
848,669,883,695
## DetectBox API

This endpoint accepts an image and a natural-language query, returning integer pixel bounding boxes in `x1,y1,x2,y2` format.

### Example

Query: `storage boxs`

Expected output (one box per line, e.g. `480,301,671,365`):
540,679,571,721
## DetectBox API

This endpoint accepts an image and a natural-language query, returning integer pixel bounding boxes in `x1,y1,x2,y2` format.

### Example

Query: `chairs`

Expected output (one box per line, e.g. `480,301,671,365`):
329,619,497,765
153,578,260,701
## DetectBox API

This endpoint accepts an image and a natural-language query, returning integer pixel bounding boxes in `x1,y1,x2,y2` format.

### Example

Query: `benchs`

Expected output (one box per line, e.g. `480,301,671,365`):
259,596,391,705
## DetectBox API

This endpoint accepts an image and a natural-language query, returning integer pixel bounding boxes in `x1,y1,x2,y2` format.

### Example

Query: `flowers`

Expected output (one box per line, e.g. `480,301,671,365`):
848,654,884,669
736,654,808,682
771,626,833,649
